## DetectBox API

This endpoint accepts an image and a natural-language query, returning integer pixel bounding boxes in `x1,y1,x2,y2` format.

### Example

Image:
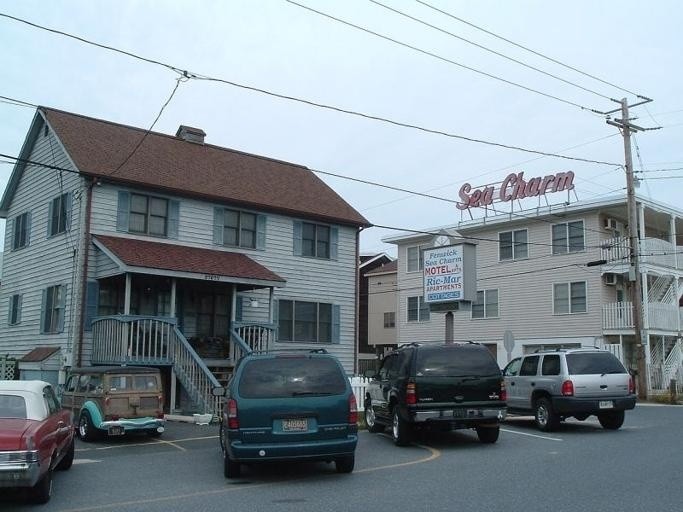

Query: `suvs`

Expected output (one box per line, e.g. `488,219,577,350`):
363,339,509,446
60,365,167,441
500,345,638,432
211,347,359,479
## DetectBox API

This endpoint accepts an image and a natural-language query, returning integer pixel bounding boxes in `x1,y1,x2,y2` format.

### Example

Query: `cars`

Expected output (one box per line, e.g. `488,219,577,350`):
0,379,76,502
